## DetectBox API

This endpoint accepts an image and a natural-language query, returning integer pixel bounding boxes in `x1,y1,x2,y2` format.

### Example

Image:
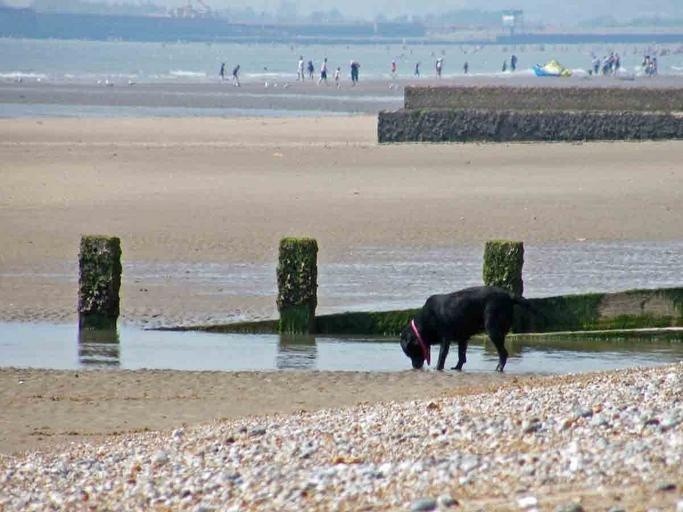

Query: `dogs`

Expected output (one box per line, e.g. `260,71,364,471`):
399,286,550,373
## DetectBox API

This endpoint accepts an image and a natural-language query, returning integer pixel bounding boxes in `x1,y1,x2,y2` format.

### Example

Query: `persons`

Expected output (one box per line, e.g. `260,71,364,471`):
390,55,518,77
295,54,359,89
231,64,239,86
590,51,660,76
217,62,225,81
